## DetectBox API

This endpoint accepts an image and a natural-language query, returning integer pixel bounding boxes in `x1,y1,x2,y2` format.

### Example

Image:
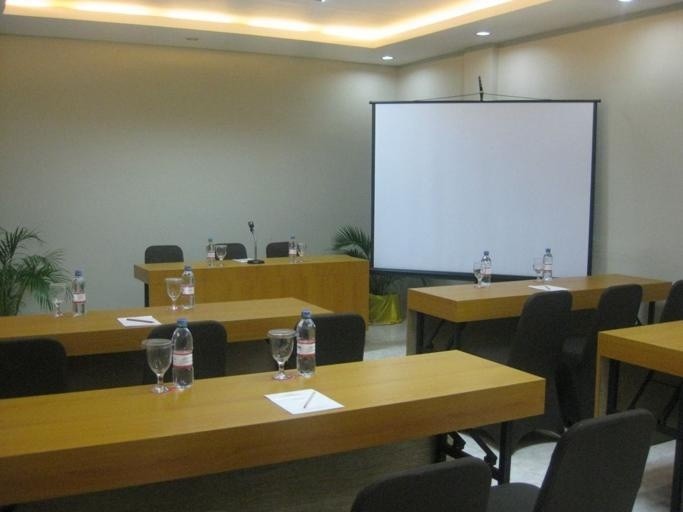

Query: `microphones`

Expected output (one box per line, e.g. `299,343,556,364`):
247,221,264,264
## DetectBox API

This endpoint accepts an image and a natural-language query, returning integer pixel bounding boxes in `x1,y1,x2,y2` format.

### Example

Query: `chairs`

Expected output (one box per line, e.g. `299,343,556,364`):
263,312,370,372
264,239,303,257
350,453,494,512
203,241,250,259
140,240,186,305
571,279,646,419
493,283,573,457
621,277,682,431
0,331,78,398
485,402,660,512
138,317,235,378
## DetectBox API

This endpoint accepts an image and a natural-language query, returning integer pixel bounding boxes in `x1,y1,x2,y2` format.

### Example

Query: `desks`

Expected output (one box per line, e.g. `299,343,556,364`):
128,254,374,330
0,296,344,368
0,344,554,511
587,312,683,512
397,270,673,376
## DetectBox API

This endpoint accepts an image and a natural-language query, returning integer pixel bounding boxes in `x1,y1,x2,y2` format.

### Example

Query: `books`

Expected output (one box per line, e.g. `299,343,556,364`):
263,387,344,415
527,284,568,291
115,315,160,326
233,258,254,264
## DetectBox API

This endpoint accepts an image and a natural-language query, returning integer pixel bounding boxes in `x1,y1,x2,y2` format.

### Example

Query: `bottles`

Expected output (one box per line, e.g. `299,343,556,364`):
170,318,194,387
180,265,194,309
480,250,491,286
543,248,552,280
288,236,296,261
205,239,215,266
70,271,85,315
295,310,315,376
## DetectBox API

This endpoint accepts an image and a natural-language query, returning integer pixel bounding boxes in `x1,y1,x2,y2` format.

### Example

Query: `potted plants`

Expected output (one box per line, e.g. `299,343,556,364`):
328,227,409,330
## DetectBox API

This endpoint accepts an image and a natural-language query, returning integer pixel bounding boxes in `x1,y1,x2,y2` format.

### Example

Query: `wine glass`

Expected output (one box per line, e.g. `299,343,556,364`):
472,261,486,289
296,240,307,262
269,330,294,380
164,278,182,312
145,338,171,395
214,245,227,267
531,257,545,282
49,282,66,320
